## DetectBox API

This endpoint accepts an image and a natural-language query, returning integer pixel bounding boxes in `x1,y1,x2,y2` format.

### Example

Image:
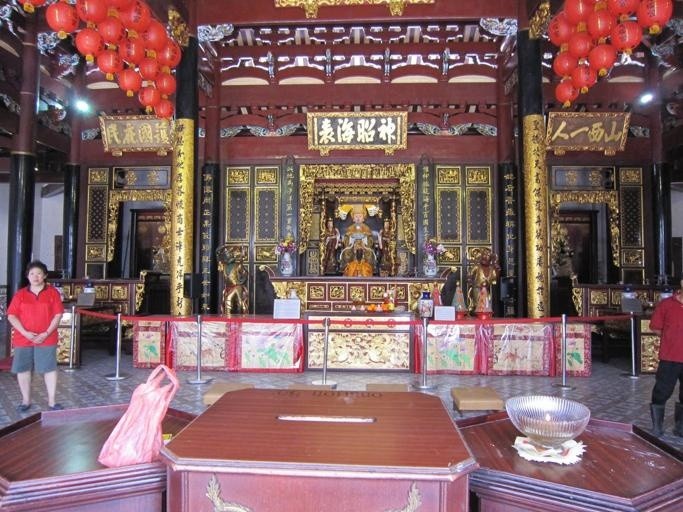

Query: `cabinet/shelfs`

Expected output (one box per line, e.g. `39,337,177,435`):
53,280,160,371
572,285,678,372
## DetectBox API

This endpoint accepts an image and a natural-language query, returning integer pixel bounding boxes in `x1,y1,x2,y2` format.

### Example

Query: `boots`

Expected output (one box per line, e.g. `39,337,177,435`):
673,403,683,437
649,404,665,438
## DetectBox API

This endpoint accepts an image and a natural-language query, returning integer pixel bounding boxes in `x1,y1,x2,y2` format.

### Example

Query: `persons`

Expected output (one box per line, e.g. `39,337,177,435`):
644,273,683,437
378,218,398,277
341,207,374,276
6,261,64,410
465,247,498,310
322,217,341,274
219,246,249,317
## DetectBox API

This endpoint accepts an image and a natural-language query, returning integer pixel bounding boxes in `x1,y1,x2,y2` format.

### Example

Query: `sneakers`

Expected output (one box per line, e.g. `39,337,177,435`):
47,403,63,410
15,404,31,412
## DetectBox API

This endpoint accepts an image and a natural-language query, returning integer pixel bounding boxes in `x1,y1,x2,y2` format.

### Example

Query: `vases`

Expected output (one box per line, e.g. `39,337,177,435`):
280,252,294,277
423,253,438,277
418,291,433,319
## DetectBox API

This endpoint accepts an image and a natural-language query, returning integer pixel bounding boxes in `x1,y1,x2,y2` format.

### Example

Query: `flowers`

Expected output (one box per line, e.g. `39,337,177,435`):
274,233,298,255
423,237,445,257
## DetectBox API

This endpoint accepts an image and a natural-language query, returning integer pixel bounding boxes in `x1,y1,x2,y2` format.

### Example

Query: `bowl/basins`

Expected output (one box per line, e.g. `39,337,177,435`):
505,394,591,456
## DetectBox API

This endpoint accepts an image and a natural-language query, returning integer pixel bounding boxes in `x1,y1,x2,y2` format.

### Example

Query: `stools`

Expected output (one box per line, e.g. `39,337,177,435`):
202,382,505,419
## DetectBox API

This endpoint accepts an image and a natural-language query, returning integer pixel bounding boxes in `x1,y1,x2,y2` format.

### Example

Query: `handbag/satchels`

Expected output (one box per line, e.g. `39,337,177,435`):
99,364,179,467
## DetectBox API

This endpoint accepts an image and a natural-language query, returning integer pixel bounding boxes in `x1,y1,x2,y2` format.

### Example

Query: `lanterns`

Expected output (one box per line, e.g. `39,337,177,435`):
546,0,675,106
18,0,182,121
